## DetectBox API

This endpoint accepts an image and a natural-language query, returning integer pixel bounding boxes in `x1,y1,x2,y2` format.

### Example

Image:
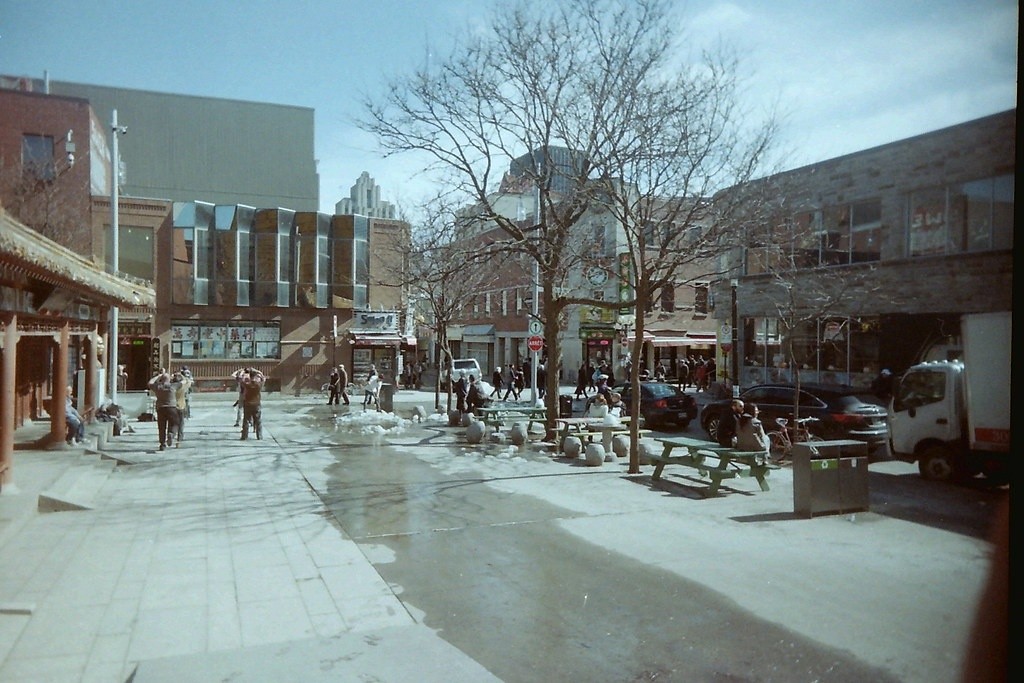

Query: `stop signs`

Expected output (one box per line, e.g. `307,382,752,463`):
527,335,543,352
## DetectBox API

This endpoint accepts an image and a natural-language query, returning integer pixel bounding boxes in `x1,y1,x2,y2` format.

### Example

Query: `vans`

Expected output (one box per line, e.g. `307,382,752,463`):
439,357,483,394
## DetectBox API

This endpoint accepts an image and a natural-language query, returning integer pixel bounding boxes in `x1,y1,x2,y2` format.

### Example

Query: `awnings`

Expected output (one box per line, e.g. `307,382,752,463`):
349,331,401,345
627,330,716,347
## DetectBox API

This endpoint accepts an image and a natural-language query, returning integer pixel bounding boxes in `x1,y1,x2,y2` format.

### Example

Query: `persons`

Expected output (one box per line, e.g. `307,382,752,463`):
65,385,91,446
466,375,486,416
360,364,384,412
339,365,349,405
625,361,650,382
231,368,266,440
871,369,892,392
490,359,546,400
576,360,616,405
452,370,467,412
654,362,666,382
585,393,626,442
677,355,716,392
403,361,426,390
326,368,341,405
148,366,194,451
717,400,772,465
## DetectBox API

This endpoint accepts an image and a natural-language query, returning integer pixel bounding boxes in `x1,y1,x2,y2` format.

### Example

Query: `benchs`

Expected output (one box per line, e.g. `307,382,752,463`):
639,449,780,475
472,415,547,421
65,405,93,423
549,428,652,437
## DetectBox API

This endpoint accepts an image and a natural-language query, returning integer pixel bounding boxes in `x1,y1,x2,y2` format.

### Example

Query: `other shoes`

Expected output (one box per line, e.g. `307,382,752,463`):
160,444,166,451
78,437,91,444
67,437,79,446
233,423,240,427
343,402,348,405
167,432,173,447
327,403,332,405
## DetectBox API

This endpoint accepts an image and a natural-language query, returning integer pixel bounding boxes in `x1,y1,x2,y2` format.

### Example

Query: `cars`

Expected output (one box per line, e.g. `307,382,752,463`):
698,379,891,458
585,380,699,433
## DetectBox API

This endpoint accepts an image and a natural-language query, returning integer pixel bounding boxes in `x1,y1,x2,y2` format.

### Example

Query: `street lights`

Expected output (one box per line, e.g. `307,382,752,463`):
729,274,740,399
515,161,540,410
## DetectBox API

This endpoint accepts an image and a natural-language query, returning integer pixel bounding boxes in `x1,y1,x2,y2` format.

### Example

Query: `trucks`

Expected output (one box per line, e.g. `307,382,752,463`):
880,309,1012,489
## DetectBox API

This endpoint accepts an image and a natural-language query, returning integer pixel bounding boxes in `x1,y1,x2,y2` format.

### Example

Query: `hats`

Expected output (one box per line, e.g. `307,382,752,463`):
597,374,609,379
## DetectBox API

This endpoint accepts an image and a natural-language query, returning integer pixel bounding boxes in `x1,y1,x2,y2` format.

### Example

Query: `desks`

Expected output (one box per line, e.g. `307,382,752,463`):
554,417,645,453
651,437,770,498
476,407,548,434
